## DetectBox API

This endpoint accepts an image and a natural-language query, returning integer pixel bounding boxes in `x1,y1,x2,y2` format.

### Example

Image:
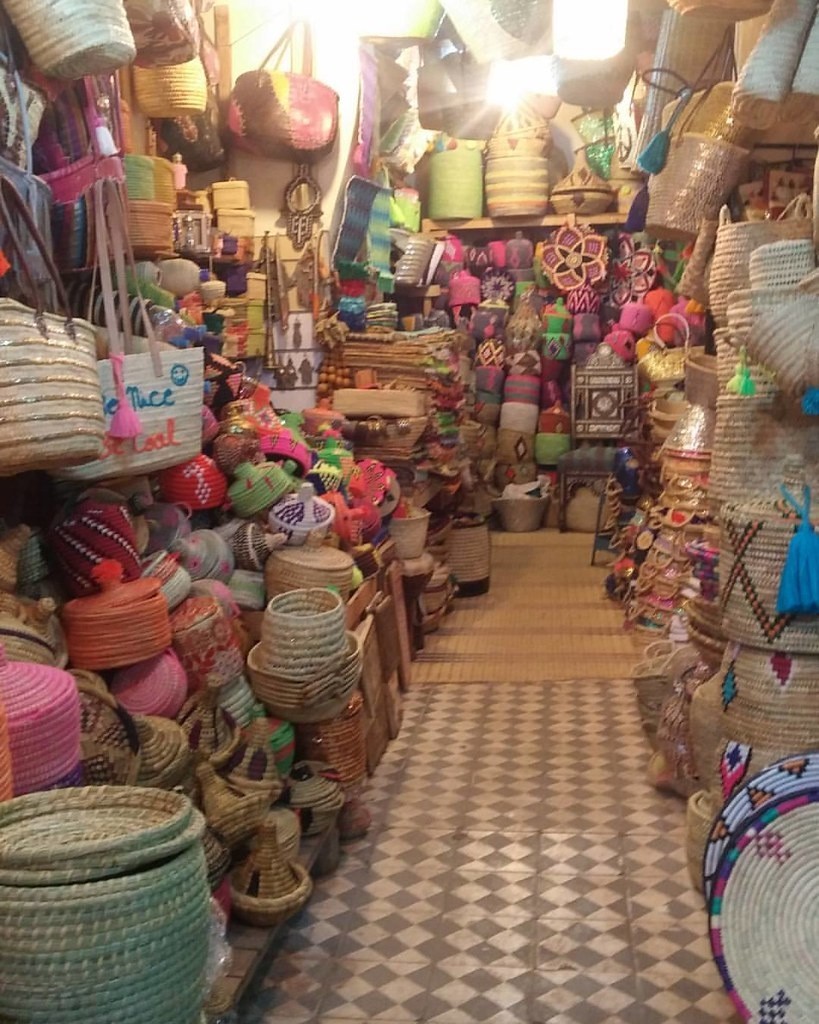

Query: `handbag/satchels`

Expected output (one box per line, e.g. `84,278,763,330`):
0,1,204,483
637,312,705,387
637,23,750,240
225,20,340,161
149,109,225,174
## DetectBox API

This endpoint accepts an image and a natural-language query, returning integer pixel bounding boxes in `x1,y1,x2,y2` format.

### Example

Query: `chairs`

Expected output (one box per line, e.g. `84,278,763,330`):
558,362,638,535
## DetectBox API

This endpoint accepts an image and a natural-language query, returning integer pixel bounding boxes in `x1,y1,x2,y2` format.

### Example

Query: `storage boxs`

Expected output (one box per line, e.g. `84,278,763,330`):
213,179,255,237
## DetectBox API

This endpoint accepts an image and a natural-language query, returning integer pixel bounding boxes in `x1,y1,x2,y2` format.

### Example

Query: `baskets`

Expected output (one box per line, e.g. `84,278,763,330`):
600,194,819,893
0,377,513,1023
